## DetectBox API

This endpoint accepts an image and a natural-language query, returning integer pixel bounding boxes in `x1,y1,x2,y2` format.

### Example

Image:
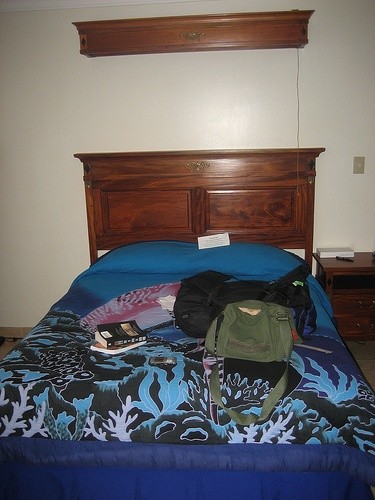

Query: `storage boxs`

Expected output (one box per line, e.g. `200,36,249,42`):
316,248,354,257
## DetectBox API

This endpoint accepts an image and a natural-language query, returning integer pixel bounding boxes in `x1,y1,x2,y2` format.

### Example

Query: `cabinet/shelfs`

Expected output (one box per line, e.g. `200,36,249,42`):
74,147,326,273
72,9,315,58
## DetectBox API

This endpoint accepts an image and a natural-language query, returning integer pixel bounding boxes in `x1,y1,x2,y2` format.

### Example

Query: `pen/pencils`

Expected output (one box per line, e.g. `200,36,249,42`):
335,256,354,262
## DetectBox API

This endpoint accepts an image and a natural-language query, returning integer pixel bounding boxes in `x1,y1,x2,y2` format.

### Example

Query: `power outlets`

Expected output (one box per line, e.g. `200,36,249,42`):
353,157,364,174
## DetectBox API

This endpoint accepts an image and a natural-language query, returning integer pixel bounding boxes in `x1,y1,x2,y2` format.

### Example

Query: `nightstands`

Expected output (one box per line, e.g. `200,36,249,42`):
312,252,375,343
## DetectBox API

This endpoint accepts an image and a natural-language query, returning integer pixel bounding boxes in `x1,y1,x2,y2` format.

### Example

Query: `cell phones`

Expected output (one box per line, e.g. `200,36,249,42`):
149,357,177,366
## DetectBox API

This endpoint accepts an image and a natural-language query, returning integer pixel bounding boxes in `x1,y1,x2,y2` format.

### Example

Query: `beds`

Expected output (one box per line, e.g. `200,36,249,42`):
0,234,375,500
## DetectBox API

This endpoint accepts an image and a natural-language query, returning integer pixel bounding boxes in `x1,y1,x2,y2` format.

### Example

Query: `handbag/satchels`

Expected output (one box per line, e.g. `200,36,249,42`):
172,264,317,341
204,300,303,426
